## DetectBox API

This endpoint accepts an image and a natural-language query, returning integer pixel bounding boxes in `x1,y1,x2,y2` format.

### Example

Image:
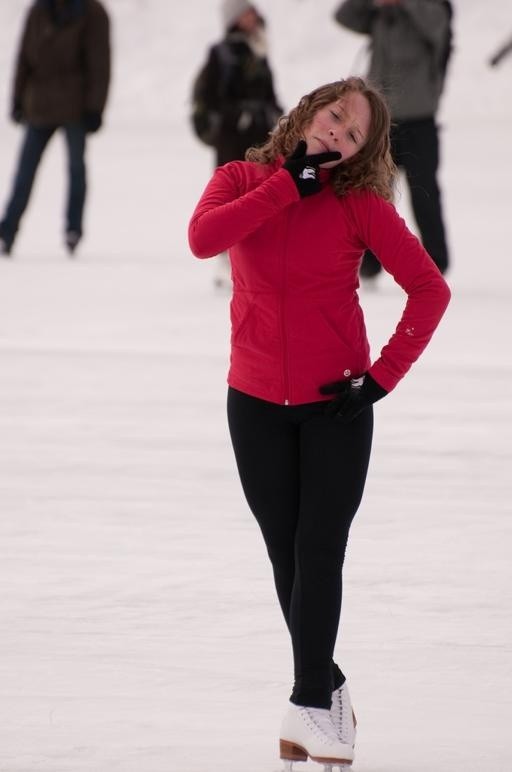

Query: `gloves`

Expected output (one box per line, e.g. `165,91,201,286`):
279,136,342,202
315,369,392,428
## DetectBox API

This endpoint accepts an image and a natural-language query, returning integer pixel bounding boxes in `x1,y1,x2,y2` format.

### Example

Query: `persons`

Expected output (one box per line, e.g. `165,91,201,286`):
187,0,283,166
334,0,456,285
186,76,455,767
0,1,112,259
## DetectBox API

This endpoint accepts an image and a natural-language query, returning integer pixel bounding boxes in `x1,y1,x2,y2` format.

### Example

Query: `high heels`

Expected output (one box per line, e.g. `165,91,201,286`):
276,695,357,764
328,675,358,751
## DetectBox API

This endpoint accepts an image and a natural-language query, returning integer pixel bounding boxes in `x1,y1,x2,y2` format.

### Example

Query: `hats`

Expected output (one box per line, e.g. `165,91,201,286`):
216,0,254,30
